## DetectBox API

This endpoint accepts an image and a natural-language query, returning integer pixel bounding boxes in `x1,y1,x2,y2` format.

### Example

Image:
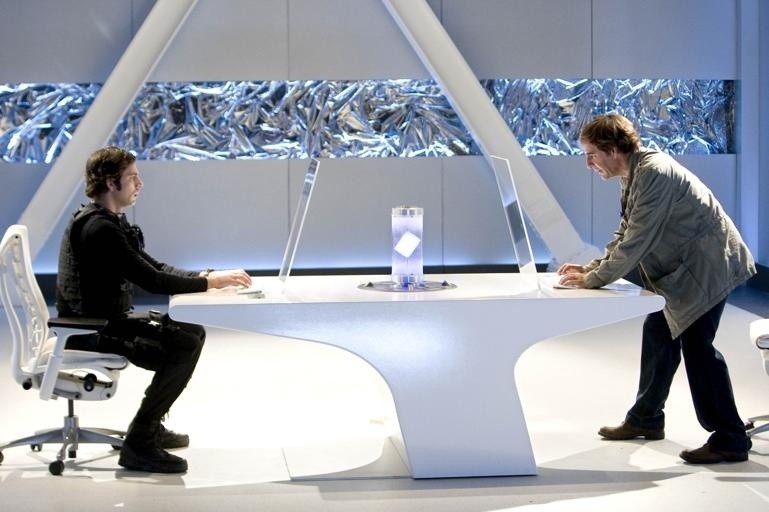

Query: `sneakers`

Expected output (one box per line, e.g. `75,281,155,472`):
157,430,191,449
118,447,188,474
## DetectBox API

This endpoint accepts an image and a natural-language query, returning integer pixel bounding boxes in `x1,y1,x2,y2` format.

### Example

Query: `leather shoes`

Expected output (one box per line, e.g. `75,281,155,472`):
597,419,665,441
678,441,750,465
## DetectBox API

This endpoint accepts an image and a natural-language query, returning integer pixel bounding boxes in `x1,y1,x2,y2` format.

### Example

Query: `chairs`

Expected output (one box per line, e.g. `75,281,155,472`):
0,221,127,476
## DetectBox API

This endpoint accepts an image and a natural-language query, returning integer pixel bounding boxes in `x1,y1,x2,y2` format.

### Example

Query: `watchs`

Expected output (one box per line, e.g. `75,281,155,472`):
203,268,216,277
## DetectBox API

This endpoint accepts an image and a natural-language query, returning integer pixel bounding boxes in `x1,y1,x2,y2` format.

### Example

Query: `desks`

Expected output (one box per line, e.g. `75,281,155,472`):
164,263,667,481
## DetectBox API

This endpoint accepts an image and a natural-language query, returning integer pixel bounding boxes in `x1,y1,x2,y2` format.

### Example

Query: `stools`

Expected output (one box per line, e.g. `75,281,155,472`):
742,318,769,450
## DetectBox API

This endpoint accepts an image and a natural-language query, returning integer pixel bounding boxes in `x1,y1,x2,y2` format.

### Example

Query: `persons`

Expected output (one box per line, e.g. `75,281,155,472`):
55,147,252,474
557,114,759,464
